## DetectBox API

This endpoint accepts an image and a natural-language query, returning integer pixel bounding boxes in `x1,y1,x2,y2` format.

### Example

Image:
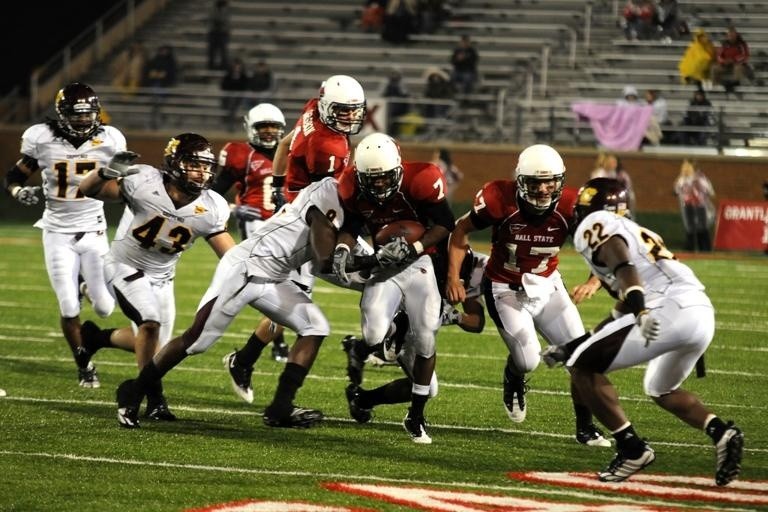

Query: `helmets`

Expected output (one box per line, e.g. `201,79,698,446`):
243,102,286,151
354,132,404,206
317,74,367,135
514,144,567,223
162,132,217,197
55,82,100,141
570,177,630,229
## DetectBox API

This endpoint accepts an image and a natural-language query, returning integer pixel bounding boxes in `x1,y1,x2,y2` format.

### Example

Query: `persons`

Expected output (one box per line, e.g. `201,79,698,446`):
110,39,145,100
565,176,744,488
144,45,176,88
79,133,237,423
207,1,230,83
363,0,457,45
213,101,297,364
424,65,452,124
219,73,370,404
4,82,128,389
450,34,480,107
621,87,638,103
114,166,360,429
677,27,753,93
446,144,612,450
341,244,490,425
676,160,716,252
618,0,690,44
428,146,462,211
644,90,675,144
382,69,413,132
591,152,637,216
334,132,456,445
247,62,271,108
677,91,711,147
220,60,245,132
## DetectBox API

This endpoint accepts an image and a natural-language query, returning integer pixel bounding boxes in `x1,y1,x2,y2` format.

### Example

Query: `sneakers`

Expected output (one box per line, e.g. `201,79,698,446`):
575,425,611,448
345,384,372,422
714,420,745,487
221,350,254,405
145,405,178,422
595,440,656,483
270,343,289,363
263,405,323,427
368,351,399,369
75,320,95,366
383,311,407,361
77,361,101,388
341,335,367,386
402,413,432,444
115,379,141,429
503,368,531,423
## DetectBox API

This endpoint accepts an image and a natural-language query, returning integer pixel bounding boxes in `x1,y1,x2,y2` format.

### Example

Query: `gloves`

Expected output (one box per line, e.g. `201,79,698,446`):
375,237,409,269
97,150,141,181
17,185,41,207
234,205,262,221
332,249,349,285
638,313,660,348
540,345,568,370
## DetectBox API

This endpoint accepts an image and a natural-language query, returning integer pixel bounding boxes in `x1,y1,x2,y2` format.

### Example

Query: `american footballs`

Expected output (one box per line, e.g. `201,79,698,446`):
375,218,427,249
